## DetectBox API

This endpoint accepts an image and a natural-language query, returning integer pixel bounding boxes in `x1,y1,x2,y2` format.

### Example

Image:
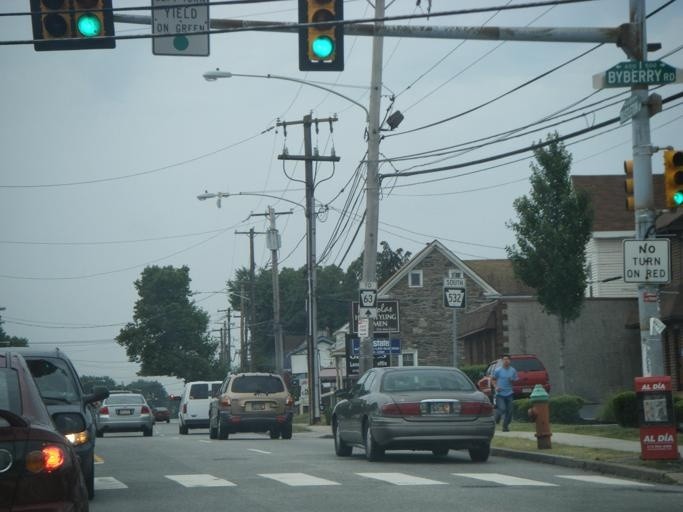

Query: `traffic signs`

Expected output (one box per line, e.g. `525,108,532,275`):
604,59,675,84
150,1,209,58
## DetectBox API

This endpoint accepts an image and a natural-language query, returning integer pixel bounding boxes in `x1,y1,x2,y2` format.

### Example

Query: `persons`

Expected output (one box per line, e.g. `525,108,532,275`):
490,354,520,432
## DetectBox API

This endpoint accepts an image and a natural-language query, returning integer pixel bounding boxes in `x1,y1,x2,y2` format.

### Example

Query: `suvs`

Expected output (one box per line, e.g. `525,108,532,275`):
209,370,294,441
476,355,550,403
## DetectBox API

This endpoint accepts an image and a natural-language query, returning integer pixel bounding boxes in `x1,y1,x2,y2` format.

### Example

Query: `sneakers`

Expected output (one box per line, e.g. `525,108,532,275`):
495,413,501,424
502,426,509,432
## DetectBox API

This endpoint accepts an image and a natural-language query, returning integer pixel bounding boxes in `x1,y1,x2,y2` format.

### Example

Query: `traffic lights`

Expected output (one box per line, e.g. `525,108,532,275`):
664,149,682,211
69,1,116,51
29,0,76,54
622,159,634,215
298,1,345,73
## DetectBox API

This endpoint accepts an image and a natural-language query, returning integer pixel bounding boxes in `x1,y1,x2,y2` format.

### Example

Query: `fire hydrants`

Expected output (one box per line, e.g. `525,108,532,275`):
523,382,555,450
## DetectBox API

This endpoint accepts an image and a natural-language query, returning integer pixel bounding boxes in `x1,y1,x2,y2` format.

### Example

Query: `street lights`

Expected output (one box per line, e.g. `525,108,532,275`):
187,289,255,375
203,69,378,372
196,189,313,428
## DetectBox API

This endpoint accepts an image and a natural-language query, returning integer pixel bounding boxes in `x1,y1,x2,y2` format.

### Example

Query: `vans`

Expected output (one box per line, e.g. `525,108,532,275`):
173,381,221,435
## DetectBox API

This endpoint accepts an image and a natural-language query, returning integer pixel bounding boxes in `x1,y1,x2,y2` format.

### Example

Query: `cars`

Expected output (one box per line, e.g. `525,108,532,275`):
328,365,496,464
0,347,170,511
